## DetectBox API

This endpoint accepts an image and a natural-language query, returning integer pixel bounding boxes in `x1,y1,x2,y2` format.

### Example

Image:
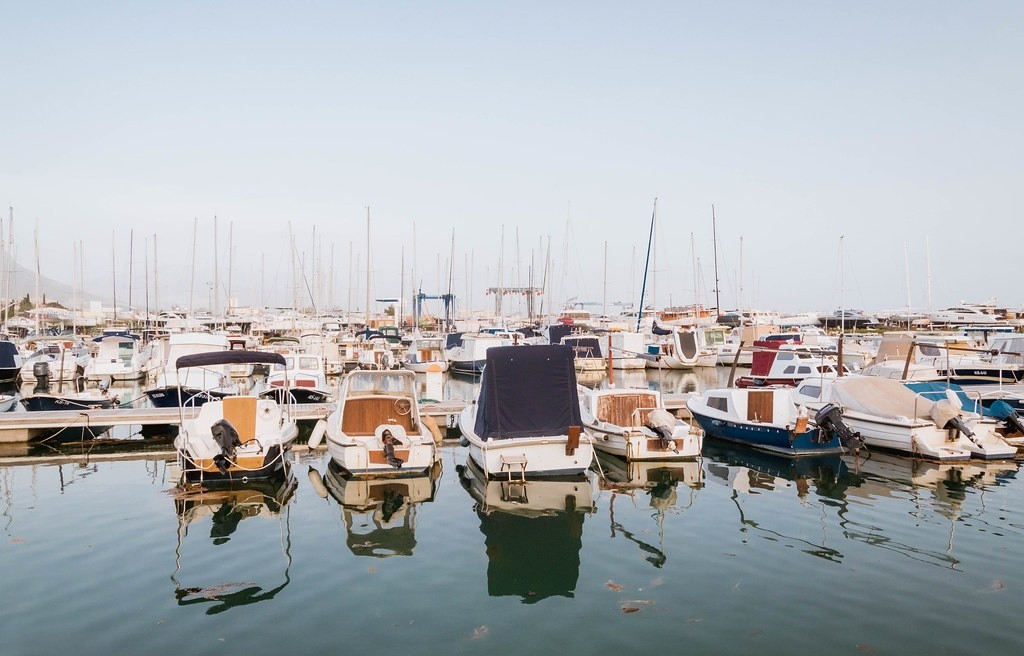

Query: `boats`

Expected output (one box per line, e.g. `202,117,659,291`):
162,461,300,616
454,456,601,604
308,457,441,557
594,458,705,570
580,389,703,459
459,345,597,483
174,350,299,483
862,446,1024,538
689,388,872,460
308,370,442,474
1,196,1024,460
702,435,865,508
